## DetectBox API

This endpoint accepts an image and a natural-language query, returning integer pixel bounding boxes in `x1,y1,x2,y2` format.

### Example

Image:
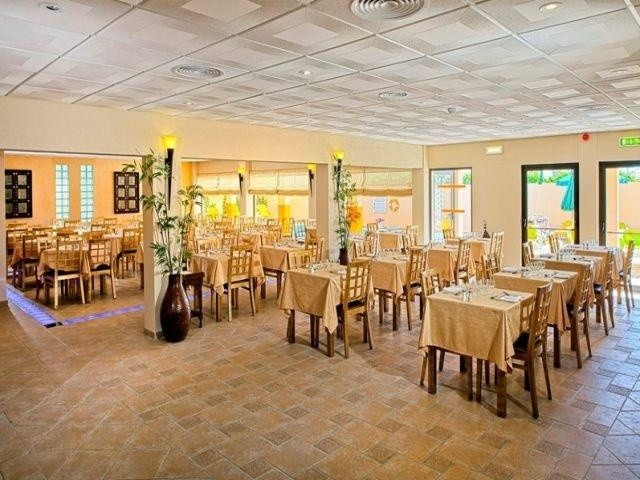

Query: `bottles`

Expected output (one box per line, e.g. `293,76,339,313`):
521,267,527,278
273,240,277,248
205,247,210,257
461,289,473,302
308,264,314,273
556,253,563,261
112,228,115,237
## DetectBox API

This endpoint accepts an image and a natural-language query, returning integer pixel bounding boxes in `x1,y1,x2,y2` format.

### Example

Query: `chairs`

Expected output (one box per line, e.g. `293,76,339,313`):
315,259,374,359
5,218,325,329
351,223,636,418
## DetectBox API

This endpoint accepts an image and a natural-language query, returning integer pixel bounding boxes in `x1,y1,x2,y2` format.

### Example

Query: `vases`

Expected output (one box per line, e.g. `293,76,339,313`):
160,274,190,342
340,248,349,265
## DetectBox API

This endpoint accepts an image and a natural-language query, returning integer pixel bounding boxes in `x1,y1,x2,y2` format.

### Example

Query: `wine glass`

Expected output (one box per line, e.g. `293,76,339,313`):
470,279,496,296
312,258,341,273
472,232,482,240
582,240,596,250
562,248,577,259
443,239,447,247
528,260,546,276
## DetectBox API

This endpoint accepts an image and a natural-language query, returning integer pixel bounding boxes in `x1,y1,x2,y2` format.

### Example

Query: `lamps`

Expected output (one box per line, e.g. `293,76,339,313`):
238,167,243,196
334,150,344,200
308,165,314,197
164,136,176,210
